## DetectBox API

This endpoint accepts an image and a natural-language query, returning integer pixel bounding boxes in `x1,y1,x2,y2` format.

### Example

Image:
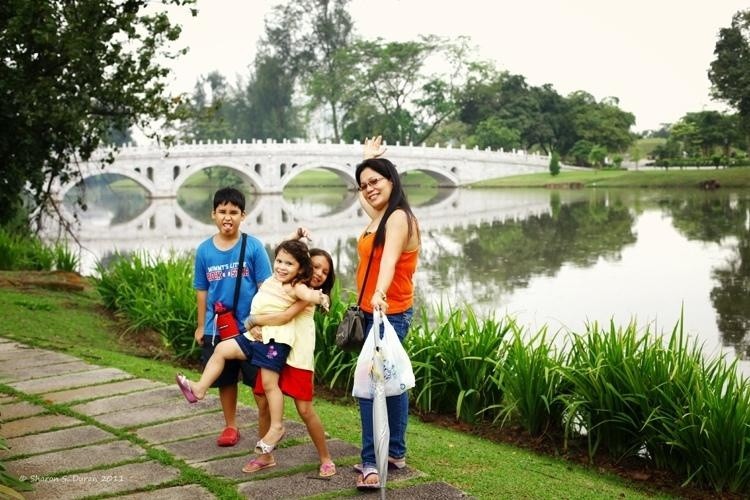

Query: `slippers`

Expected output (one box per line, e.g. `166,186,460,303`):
254,431,286,455
353,462,406,475
175,373,198,404
320,462,335,476
217,426,240,446
356,467,381,491
242,458,276,473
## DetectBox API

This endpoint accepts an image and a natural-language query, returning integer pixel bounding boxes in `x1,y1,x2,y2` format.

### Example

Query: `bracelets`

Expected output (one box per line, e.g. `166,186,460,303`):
376,288,387,299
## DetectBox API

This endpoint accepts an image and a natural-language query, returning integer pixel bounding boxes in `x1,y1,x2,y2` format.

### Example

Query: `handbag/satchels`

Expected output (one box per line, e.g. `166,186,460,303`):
335,236,377,352
211,233,249,340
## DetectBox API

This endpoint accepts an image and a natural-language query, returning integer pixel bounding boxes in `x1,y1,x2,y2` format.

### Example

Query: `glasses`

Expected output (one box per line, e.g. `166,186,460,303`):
357,178,385,191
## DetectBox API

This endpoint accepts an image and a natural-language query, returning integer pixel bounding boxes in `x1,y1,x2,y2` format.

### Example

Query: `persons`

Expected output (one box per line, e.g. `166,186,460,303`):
354,135,421,493
240,249,336,476
193,187,273,448
176,229,330,454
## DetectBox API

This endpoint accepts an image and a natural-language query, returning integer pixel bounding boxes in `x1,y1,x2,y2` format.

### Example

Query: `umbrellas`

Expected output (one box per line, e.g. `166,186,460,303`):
371,308,390,500
